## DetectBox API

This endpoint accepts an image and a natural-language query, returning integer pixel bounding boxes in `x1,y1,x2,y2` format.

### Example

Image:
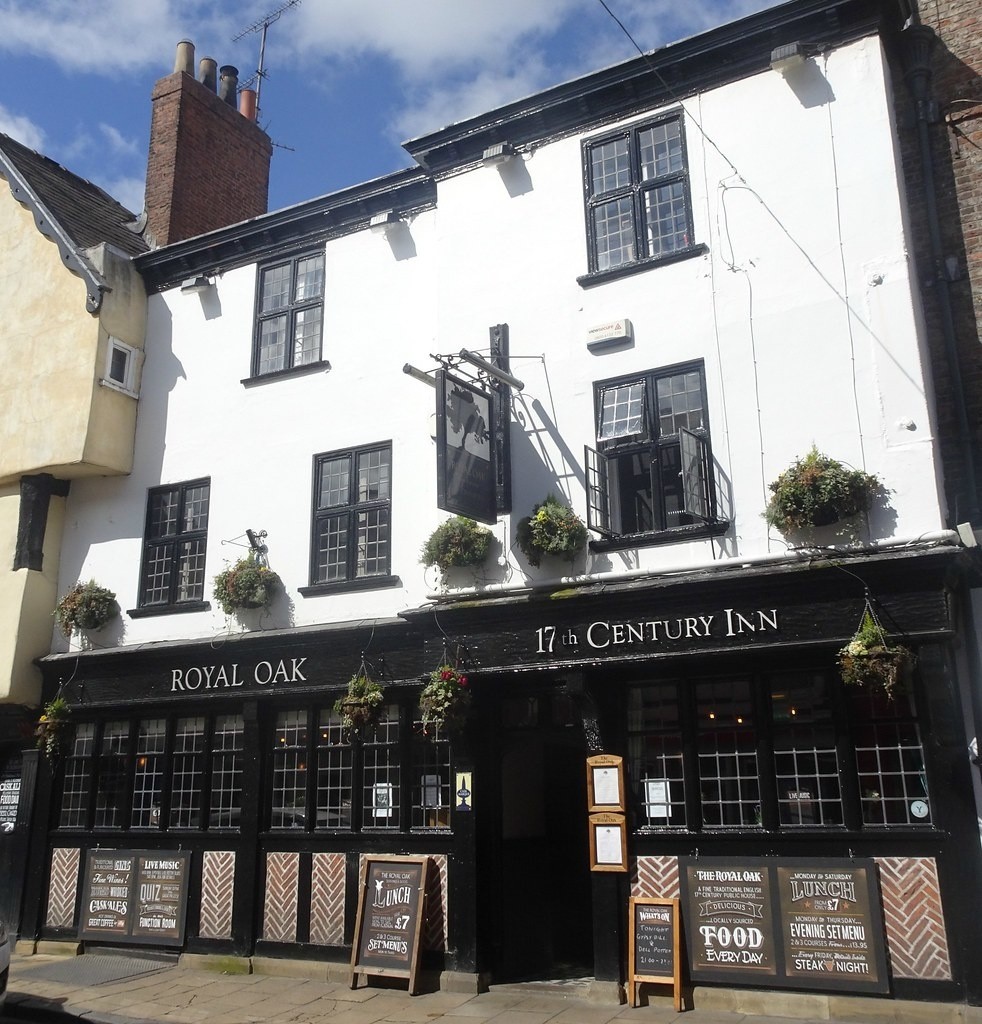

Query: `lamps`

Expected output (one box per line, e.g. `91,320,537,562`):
481,140,519,168
769,41,810,74
180,273,211,293
368,208,402,231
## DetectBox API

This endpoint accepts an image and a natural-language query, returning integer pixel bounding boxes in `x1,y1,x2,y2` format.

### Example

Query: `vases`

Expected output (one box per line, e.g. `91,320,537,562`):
419,694,473,733
839,652,902,687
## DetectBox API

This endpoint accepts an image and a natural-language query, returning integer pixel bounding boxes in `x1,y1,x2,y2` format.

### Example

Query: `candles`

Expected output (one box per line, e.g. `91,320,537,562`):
53,582,121,631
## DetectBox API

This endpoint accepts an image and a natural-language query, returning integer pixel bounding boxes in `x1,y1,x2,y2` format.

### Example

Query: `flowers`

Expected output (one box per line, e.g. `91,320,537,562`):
420,663,472,693
834,612,915,703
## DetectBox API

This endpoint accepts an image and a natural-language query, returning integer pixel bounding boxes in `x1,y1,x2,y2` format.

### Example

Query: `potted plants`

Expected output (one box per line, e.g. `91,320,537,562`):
758,444,879,534
516,493,588,570
35,698,72,754
419,514,493,575
334,674,385,725
213,559,282,615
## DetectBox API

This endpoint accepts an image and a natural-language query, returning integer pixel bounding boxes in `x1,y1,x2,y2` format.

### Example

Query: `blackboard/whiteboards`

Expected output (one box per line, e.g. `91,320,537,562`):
350,856,430,979
627,895,682,984
